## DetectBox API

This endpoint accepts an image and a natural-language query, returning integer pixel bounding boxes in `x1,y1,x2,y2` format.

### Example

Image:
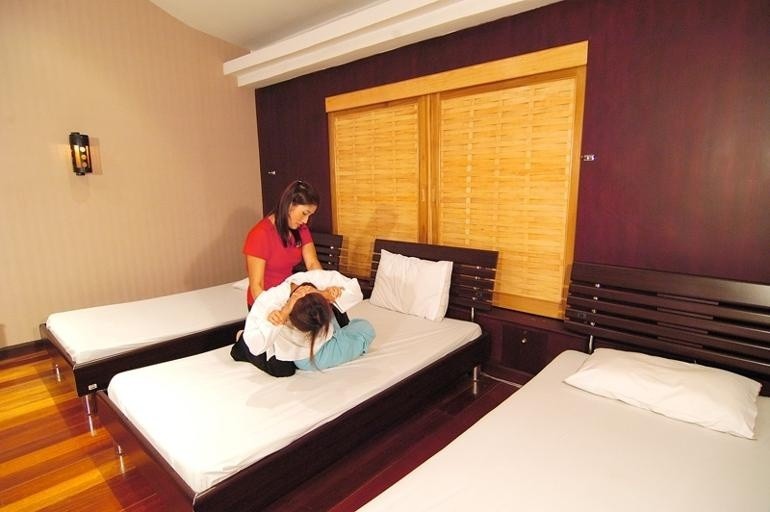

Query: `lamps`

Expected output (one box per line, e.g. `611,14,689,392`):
68,130,93,177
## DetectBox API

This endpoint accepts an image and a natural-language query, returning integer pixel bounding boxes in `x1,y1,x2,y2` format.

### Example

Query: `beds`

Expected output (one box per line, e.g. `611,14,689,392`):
350,260,769,512
38,232,345,416
89,238,499,512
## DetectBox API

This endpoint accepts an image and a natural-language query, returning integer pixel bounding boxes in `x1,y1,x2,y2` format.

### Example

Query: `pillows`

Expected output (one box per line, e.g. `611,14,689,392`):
370,245,454,324
565,344,764,442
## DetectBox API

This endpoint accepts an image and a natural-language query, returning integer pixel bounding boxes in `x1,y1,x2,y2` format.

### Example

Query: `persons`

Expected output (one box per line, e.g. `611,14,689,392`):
243,269,376,370
230,178,344,378
